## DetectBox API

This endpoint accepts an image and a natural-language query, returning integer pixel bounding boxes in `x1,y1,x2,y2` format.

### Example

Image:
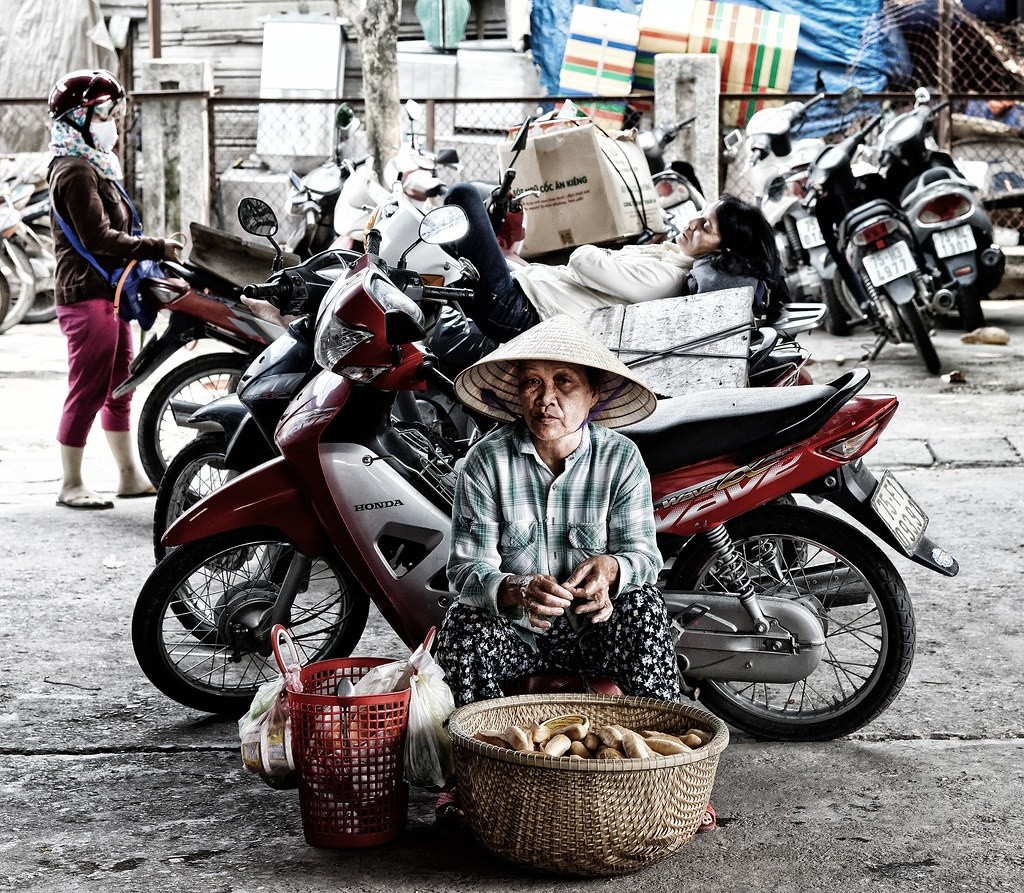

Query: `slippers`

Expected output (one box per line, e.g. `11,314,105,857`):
435,760,465,822
697,804,716,832
117,486,158,498
56,495,114,510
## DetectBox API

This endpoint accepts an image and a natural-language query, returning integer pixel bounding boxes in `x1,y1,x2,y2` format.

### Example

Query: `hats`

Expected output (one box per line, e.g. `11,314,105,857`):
453,313,657,430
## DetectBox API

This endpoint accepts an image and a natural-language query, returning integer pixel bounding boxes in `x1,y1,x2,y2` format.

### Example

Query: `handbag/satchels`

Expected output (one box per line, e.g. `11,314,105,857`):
111,259,166,331
239,664,303,788
408,641,457,788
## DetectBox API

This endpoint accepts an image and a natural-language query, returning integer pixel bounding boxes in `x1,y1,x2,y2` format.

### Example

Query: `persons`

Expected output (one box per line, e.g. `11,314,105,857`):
443,184,776,341
432,314,716,830
47,68,184,509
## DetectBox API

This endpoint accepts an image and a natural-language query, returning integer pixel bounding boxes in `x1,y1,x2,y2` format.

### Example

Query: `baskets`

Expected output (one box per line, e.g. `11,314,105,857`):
442,693,729,873
272,624,438,847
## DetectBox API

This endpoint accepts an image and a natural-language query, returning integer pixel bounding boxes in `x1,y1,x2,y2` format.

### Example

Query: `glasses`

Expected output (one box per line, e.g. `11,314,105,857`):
94,110,121,123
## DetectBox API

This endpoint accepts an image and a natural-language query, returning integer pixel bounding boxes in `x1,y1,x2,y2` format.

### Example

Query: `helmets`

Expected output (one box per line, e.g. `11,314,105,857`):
48,69,128,121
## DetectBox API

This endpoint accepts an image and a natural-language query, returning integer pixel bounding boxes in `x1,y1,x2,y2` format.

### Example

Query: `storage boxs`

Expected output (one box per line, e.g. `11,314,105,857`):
394,36,545,184
497,117,665,258
219,14,345,244
556,0,801,131
